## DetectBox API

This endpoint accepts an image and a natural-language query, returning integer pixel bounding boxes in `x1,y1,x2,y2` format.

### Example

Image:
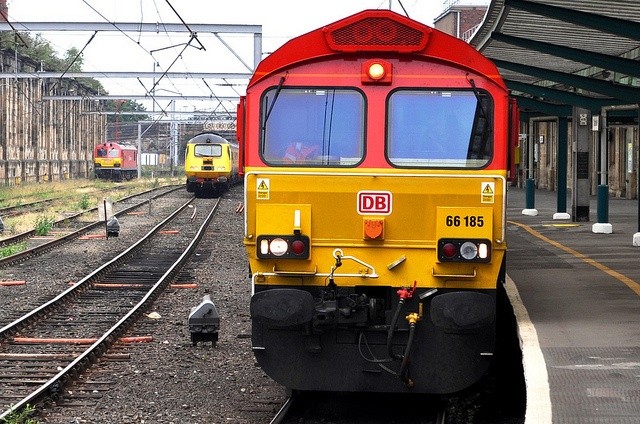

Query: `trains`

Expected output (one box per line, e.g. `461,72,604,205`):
93,142,138,182
236,9,525,411
184,133,240,199
